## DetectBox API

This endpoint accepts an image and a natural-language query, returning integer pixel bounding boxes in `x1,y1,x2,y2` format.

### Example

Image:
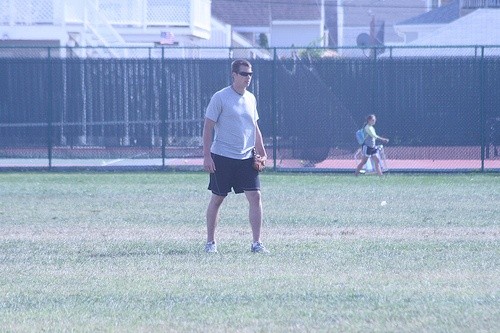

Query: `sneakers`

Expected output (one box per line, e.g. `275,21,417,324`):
204,241,217,253
251,241,270,253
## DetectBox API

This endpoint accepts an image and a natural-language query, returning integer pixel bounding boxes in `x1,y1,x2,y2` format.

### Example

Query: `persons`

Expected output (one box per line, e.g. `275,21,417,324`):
354,114,390,177
203,59,271,255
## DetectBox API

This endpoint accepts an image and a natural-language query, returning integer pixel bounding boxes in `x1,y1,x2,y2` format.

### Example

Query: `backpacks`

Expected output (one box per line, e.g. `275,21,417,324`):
356,128,368,145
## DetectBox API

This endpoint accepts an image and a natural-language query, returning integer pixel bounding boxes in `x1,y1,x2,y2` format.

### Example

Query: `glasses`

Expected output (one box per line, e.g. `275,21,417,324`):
238,72,253,76
